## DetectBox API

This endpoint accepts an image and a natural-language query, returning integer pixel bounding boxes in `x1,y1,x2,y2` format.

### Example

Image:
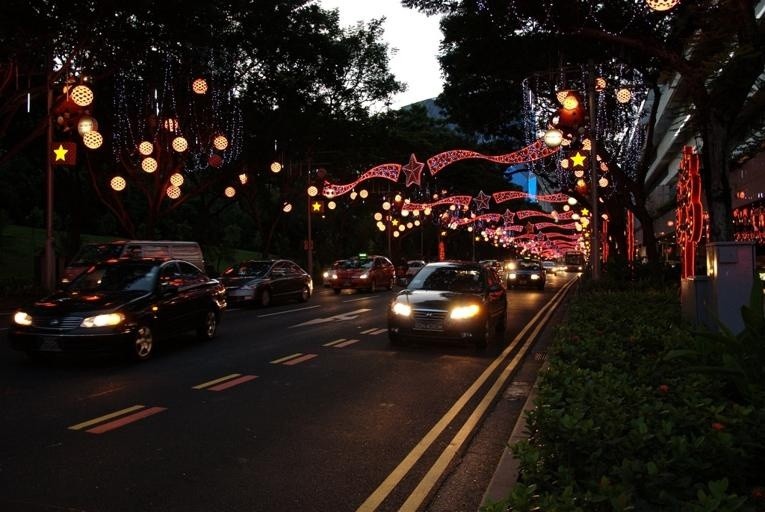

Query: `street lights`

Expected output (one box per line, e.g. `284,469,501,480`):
557,62,600,280
382,189,403,260
307,164,319,281
45,80,102,295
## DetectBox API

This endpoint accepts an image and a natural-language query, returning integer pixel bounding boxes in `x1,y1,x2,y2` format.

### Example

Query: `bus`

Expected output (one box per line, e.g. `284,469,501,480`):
565,251,585,272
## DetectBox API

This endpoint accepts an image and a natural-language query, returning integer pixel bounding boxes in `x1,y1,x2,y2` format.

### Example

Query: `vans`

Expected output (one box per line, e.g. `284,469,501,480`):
58,239,206,287
